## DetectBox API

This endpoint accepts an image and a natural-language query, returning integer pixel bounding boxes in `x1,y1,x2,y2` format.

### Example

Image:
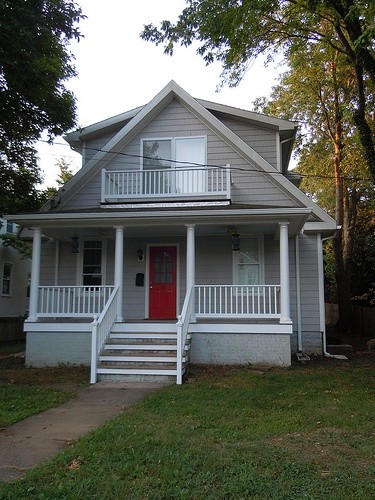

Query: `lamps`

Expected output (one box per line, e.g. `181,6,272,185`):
70,230,80,254
231,225,241,252
